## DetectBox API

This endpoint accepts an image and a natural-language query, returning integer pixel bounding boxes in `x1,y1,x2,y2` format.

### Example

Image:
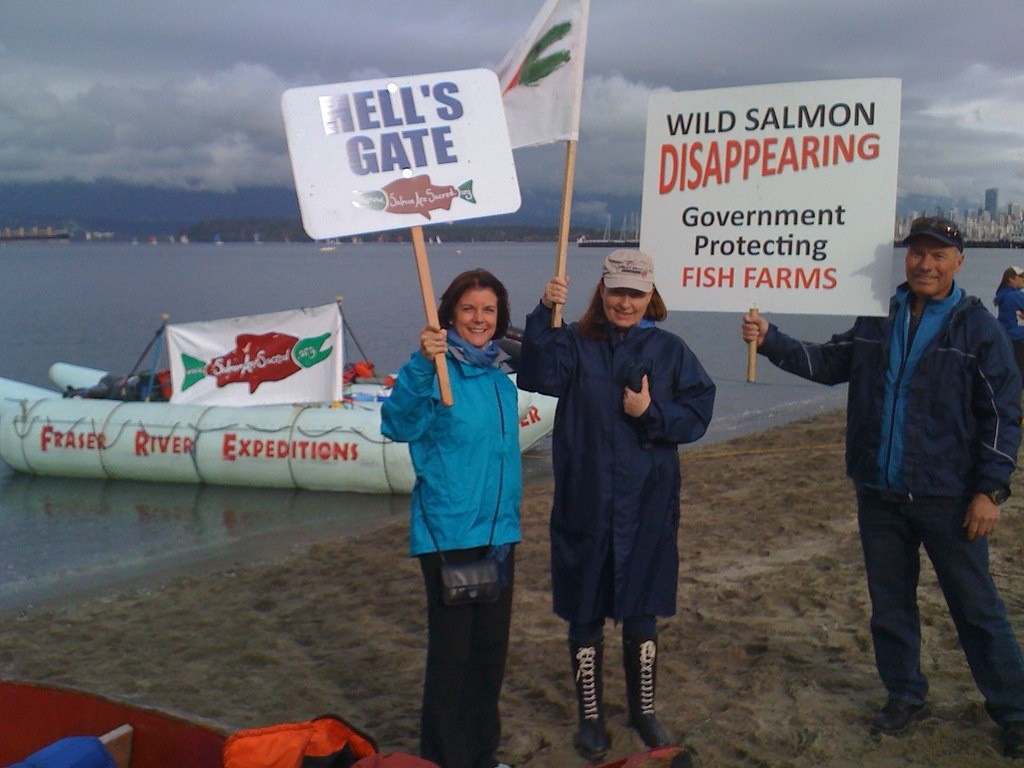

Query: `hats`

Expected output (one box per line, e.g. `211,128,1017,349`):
1003,266,1024,281
903,216,963,254
601,248,654,293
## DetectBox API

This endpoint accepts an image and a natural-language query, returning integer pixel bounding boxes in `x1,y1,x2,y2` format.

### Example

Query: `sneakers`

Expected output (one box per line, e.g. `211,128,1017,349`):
872,700,926,736
1003,723,1024,758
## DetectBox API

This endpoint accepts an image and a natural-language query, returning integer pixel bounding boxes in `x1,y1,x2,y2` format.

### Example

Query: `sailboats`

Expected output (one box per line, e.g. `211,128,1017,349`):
576,210,641,248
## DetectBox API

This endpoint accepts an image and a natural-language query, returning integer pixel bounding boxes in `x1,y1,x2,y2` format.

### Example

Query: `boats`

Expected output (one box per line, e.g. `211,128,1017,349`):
0,674,443,768
0,298,565,496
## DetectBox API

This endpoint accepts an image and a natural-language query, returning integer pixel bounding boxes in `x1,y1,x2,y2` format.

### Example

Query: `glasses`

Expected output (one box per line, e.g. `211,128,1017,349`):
910,217,962,243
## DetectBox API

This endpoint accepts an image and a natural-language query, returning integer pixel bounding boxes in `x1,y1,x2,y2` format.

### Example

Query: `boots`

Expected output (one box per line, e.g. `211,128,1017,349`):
568,638,608,759
623,633,668,747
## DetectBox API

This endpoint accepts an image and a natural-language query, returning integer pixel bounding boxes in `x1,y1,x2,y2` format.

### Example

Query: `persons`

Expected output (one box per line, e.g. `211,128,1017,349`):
518,249,717,762
380,269,524,768
743,218,1024,758
993,266,1024,388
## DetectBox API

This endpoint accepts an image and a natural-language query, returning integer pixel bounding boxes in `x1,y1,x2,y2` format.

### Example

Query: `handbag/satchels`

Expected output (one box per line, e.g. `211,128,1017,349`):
442,558,502,611
